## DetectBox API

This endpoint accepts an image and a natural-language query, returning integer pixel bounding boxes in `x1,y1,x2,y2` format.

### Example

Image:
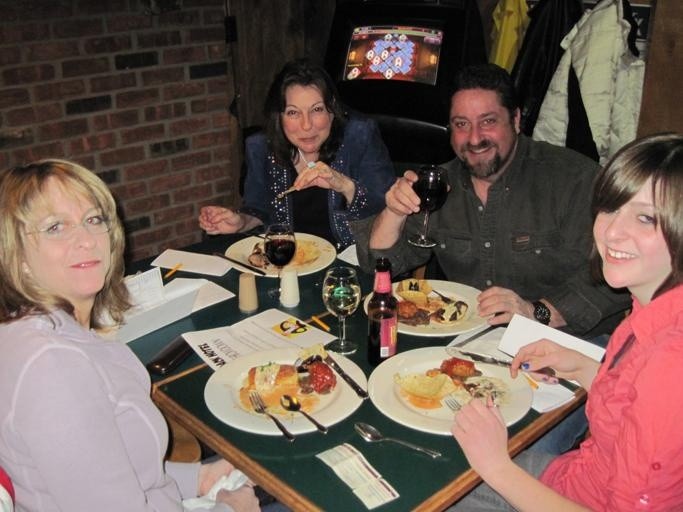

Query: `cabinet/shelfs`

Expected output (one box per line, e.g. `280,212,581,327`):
238,272,260,314
276,265,300,310
365,255,412,362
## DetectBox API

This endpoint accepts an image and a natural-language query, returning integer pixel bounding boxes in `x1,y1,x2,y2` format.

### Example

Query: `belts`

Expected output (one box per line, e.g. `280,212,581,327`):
319,267,361,355
261,224,295,298
405,164,447,250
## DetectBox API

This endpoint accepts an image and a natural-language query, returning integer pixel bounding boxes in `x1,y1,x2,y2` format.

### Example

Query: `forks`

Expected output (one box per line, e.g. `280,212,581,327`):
438,390,462,413
245,390,294,445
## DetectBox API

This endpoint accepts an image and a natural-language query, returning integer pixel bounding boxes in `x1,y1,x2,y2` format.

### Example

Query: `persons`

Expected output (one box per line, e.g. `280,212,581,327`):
196,61,400,256
450,132,682,510
350,64,634,347
0,156,263,512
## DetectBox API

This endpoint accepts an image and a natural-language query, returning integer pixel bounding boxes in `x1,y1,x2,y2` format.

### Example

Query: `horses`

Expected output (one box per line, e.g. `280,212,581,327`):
146,336,194,376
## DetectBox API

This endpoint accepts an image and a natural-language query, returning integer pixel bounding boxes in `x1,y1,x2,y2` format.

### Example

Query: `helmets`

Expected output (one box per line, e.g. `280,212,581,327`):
203,348,366,437
367,346,535,437
225,229,339,279
363,279,496,338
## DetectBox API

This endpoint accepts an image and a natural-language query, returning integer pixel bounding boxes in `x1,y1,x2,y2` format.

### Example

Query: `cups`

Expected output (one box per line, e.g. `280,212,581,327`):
25,206,109,239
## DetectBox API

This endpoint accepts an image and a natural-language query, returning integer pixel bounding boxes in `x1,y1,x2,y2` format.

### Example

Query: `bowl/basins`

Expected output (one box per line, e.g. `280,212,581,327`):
295,148,319,167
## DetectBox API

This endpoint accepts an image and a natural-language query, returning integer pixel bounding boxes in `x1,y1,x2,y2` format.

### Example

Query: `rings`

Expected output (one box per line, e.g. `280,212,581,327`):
522,368,538,389
163,262,184,279
311,316,331,333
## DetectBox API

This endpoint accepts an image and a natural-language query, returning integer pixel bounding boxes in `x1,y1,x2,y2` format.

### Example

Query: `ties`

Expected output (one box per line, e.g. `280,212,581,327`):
324,9,488,129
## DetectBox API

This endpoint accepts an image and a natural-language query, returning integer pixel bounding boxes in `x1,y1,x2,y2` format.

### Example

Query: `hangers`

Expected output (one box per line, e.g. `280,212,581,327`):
279,393,331,434
350,420,442,461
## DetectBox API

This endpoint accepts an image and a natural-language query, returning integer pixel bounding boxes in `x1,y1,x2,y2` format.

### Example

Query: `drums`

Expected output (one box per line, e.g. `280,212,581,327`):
440,346,556,377
214,252,266,276
321,353,368,400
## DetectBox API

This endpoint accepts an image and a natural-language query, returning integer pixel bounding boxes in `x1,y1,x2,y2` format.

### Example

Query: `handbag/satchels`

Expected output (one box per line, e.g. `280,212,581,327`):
235,211,246,232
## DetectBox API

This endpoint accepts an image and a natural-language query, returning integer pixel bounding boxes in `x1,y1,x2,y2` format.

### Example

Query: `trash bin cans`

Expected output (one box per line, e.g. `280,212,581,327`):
532,301,551,325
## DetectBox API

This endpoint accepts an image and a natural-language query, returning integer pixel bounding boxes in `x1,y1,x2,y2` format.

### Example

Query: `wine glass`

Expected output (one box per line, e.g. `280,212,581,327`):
486,402,499,409
307,163,314,168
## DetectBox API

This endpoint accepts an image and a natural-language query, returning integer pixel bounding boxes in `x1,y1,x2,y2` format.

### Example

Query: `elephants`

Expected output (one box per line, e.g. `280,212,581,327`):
447,356,474,378
310,361,337,393
397,301,418,319
